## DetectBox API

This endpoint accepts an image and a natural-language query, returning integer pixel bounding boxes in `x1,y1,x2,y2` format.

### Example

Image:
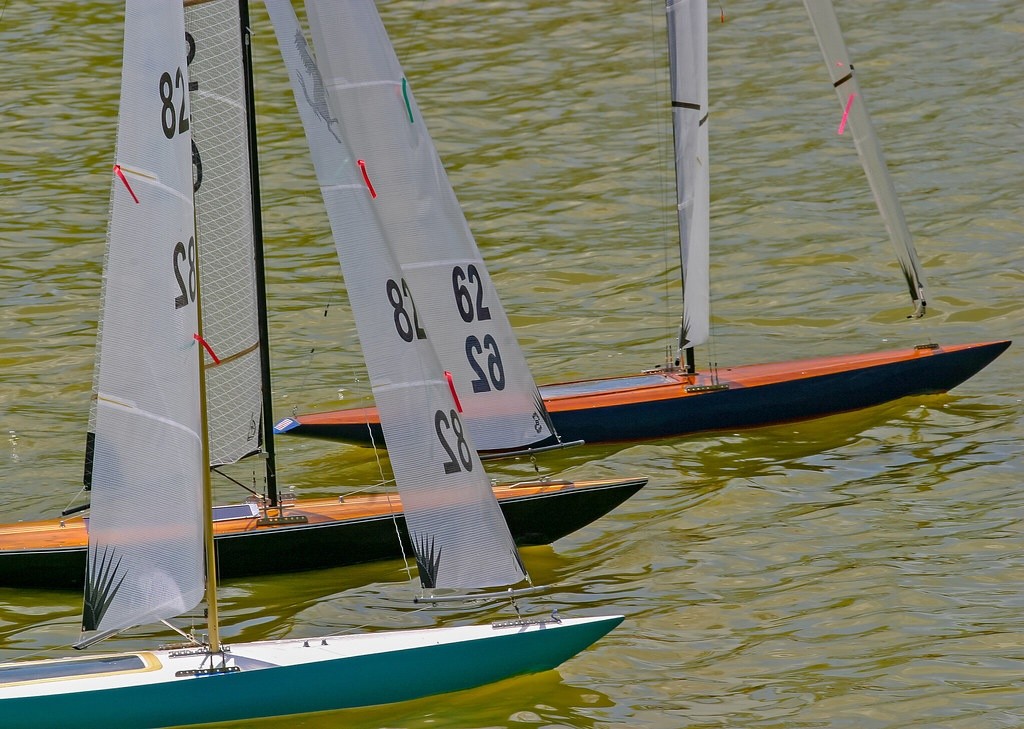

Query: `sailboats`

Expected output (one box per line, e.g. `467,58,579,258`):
0,0,626,729
0,0,642,578
271,1,1012,453
